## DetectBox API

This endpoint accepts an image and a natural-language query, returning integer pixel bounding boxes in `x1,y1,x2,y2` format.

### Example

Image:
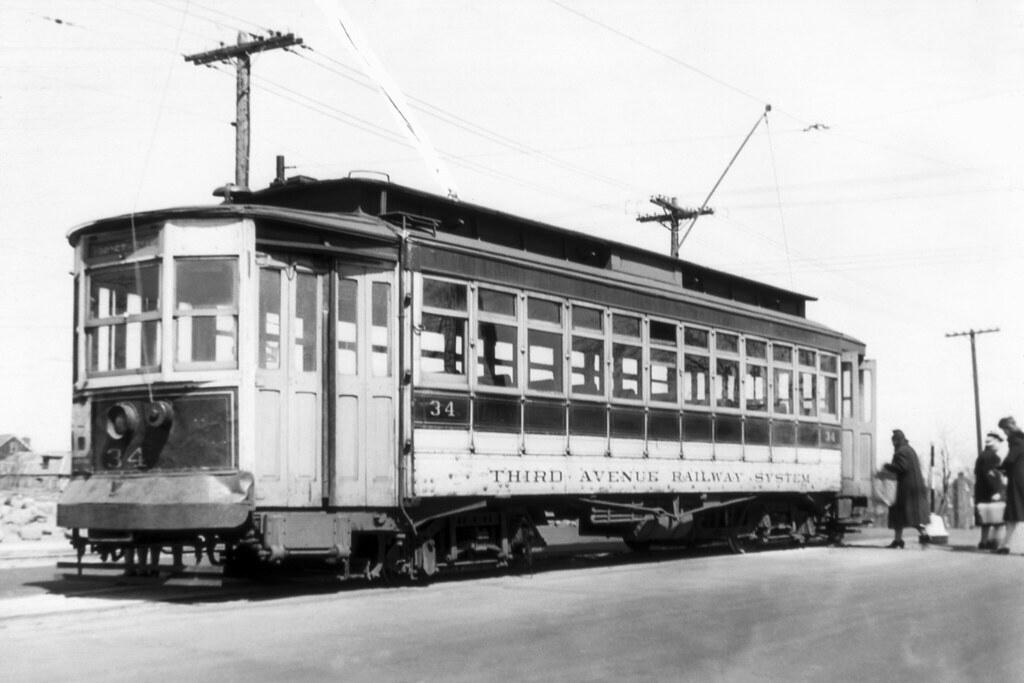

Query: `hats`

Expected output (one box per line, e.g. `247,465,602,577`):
985,433,1003,447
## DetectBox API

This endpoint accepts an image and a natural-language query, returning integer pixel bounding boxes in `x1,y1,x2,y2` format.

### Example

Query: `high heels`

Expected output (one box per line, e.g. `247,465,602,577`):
919,534,935,546
886,539,905,549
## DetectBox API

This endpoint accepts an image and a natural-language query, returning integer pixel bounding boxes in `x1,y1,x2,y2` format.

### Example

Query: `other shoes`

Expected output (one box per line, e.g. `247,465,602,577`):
997,547,1010,555
978,539,997,551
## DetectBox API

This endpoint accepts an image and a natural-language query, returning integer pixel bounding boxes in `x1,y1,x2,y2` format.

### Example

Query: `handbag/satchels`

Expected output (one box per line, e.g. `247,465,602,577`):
870,467,898,507
976,497,1006,523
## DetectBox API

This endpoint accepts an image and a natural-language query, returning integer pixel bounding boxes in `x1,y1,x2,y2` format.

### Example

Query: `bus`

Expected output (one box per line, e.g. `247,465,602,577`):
53,104,882,587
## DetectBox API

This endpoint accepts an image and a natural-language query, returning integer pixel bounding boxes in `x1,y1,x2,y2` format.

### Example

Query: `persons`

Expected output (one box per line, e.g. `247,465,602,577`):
884,429,931,549
973,433,1002,549
992,416,1024,554
